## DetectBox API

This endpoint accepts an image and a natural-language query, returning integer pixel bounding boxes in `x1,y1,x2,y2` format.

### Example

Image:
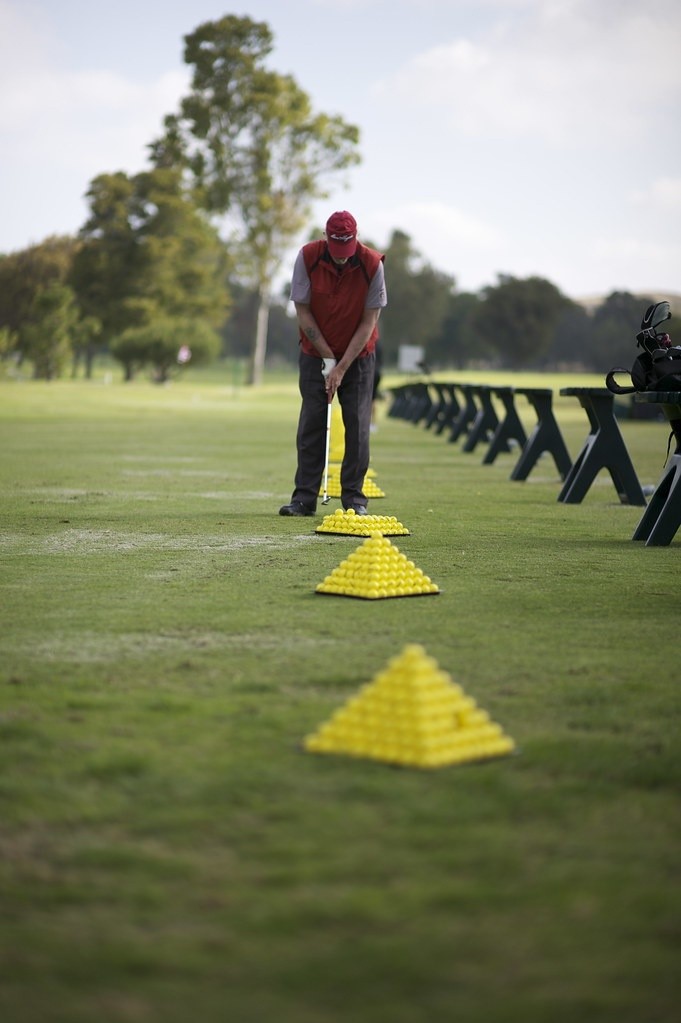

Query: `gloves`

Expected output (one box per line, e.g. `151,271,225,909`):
321,357,336,394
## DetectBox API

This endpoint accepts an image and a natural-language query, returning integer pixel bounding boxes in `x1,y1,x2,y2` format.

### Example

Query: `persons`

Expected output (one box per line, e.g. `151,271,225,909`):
279,209,386,516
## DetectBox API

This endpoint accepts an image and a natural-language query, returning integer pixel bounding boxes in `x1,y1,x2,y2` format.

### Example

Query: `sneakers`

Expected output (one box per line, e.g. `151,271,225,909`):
347,500,366,516
280,500,316,517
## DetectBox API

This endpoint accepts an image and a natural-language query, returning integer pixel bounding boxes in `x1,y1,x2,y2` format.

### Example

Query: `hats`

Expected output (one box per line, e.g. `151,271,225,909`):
326,210,357,257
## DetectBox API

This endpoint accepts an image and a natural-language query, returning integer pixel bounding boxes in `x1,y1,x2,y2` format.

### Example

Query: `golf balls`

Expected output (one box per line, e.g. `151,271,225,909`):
308,399,389,498
299,644,517,770
315,509,441,599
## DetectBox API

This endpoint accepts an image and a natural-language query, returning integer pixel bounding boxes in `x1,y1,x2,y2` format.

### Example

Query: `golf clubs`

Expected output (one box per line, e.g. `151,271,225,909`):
321,386,333,505
